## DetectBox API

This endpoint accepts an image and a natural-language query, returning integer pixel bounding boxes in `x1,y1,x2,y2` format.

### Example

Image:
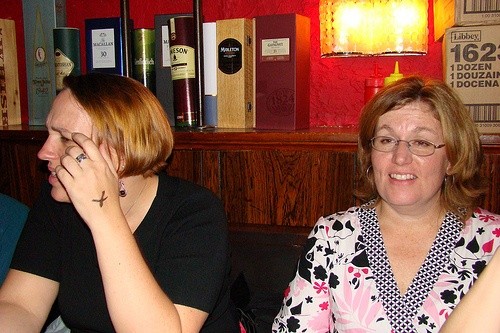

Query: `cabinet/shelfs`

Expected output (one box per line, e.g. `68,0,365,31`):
0,123,500,231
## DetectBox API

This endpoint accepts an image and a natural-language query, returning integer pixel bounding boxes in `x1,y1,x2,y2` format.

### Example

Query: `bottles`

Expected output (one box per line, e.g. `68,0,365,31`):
365,59,405,106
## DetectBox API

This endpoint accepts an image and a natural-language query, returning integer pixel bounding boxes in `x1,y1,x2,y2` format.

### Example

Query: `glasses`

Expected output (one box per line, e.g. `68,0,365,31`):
370,135,445,157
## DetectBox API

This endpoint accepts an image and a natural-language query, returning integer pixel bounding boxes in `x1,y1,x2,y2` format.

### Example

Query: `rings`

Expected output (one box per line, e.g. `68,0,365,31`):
75,153,88,164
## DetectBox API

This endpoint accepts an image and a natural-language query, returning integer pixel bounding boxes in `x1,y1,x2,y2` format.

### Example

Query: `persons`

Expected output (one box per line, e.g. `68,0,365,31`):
0,74,256,333
271,77,500,333
438,247,500,333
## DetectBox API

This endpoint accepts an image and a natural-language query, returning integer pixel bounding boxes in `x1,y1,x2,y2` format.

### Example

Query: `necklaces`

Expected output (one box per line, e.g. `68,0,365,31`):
123,178,148,217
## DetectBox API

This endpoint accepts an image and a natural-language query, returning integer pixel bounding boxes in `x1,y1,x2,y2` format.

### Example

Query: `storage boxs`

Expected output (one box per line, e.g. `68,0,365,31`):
443,25,500,134
216,18,252,128
455,0,500,26
154,13,197,124
252,13,310,130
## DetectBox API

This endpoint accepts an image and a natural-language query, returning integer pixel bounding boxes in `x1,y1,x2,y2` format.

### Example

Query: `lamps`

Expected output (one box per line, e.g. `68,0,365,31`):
318,0,429,57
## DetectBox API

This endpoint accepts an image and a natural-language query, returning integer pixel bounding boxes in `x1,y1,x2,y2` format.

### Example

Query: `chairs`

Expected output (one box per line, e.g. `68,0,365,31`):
223,223,315,333
